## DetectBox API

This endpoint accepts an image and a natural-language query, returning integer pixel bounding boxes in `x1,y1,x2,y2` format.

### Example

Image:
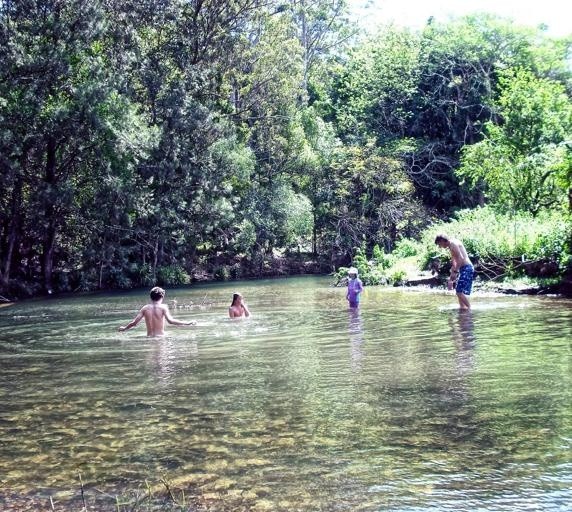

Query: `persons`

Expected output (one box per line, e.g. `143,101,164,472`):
228,293,250,318
349,308,362,336
448,309,475,373
345,267,363,307
119,286,196,337
435,234,474,308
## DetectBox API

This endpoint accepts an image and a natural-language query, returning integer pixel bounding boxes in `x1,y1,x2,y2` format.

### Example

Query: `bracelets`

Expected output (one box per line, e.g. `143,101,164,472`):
453,270,457,273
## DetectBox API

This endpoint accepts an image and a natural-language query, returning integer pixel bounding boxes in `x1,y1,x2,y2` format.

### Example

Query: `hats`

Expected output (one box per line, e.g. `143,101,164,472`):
347,268,359,274
